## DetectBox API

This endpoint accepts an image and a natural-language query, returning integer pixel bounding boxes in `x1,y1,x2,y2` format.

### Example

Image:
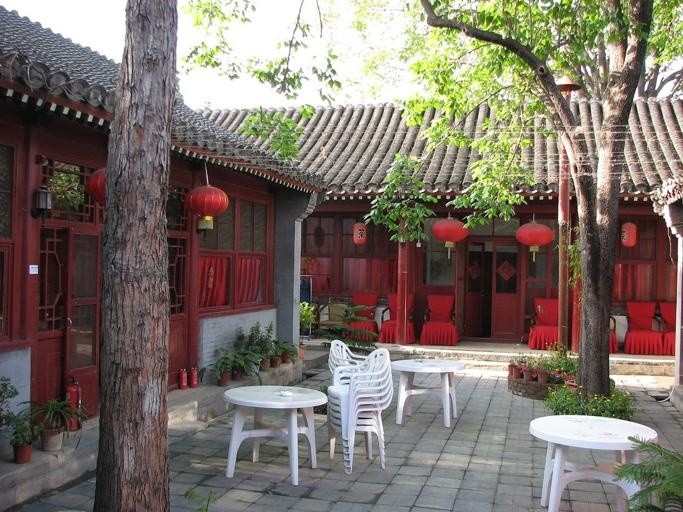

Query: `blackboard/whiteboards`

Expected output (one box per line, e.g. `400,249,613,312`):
299,275,312,336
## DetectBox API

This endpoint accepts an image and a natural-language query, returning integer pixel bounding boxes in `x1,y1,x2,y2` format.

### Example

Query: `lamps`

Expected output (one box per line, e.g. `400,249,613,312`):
31,184,53,228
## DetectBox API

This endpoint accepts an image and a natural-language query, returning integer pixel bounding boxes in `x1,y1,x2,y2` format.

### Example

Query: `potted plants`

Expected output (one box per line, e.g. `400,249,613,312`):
0,375,19,458
3,409,42,464
299,301,317,345
17,398,90,452
199,321,299,386
508,341,552,384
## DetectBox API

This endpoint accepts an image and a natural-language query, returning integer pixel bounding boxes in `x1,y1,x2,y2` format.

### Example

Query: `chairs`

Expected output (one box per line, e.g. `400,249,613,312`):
529,298,558,349
341,292,378,341
608,315,620,353
327,338,394,476
658,301,676,355
380,293,416,344
626,301,662,355
419,294,458,345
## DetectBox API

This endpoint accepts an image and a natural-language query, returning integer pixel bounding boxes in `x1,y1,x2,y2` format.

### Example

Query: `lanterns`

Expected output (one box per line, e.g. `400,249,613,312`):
84,168,107,207
515,212,554,264
183,162,229,231
619,221,638,249
431,212,470,260
352,222,369,246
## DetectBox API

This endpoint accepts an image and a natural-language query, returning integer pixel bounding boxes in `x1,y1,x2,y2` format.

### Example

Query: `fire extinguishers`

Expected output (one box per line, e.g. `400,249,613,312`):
190,368,197,388
177,368,187,389
66,375,82,431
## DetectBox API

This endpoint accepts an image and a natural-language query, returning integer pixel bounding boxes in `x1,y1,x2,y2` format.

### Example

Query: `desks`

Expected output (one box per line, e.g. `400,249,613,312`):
528,414,659,512
390,359,466,428
223,385,329,486
